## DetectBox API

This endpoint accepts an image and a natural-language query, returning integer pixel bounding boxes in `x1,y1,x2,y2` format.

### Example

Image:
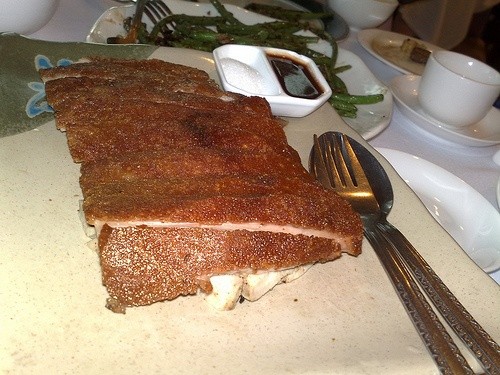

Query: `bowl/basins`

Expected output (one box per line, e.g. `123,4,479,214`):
0,0,60,37
323,0,400,34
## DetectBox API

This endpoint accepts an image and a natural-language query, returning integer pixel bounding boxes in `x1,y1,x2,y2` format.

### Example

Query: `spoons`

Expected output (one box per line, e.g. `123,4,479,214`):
306,130,500,375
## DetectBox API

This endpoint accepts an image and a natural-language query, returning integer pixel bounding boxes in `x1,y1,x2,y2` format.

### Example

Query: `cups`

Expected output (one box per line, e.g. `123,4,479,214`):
418,49,500,129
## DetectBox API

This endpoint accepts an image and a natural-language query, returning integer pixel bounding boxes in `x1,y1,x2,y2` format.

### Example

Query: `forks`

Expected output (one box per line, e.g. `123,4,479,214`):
116,0,177,37
311,133,475,375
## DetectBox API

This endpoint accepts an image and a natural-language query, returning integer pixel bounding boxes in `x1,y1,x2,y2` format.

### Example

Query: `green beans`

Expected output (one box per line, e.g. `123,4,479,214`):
105,0,385,118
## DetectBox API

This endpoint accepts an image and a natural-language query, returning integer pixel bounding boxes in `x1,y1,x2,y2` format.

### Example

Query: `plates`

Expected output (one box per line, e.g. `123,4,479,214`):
213,44,332,118
357,28,448,76
85,0,394,143
389,74,500,148
234,0,325,31
